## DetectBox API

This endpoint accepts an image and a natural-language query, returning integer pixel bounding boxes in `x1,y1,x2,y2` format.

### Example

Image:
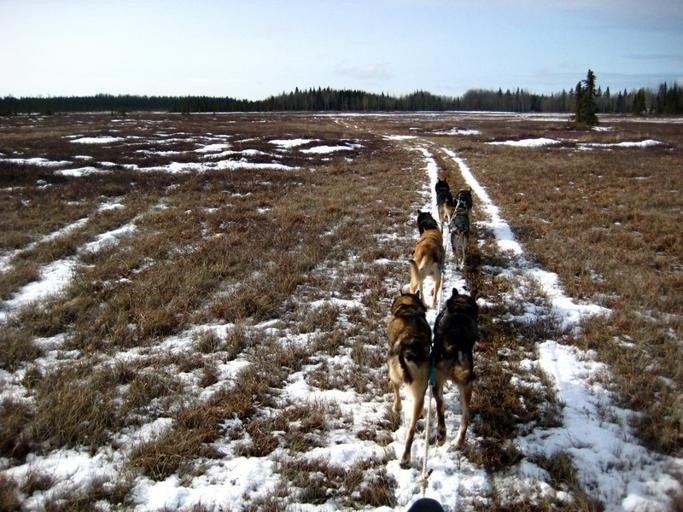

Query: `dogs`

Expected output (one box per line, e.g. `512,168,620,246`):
435,177,455,233
430,286,481,451
386,289,432,468
448,187,473,271
409,209,445,309
